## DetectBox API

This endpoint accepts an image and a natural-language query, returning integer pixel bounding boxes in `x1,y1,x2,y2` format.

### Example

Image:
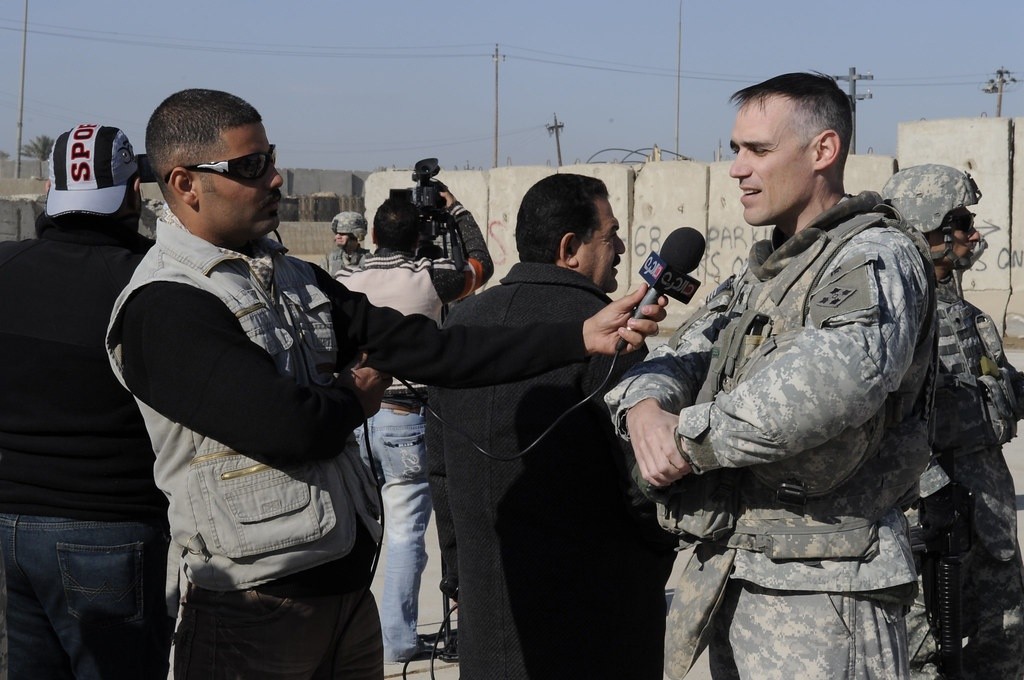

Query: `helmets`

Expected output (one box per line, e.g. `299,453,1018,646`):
881,164,982,234
330,211,368,234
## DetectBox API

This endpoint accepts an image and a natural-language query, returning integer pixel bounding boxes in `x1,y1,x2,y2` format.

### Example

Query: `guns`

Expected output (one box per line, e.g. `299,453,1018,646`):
909,491,971,680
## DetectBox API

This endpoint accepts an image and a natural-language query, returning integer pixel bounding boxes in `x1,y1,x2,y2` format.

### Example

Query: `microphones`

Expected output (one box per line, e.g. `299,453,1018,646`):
616,227,705,352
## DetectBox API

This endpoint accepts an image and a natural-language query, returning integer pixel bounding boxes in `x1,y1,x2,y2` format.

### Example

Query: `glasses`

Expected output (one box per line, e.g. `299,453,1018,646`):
164,143,278,184
951,212,976,234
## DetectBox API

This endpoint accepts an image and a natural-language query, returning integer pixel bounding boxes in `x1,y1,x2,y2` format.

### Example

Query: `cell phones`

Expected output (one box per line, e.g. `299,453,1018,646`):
135,154,157,183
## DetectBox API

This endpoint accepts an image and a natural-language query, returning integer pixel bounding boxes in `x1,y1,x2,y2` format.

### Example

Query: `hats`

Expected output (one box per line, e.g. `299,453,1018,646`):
44,123,139,218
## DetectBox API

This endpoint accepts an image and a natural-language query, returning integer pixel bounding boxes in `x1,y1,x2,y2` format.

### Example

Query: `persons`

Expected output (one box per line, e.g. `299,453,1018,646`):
423,173,649,680
603,70,940,680
319,211,375,452
332,178,495,660
880,164,1024,679
0,124,156,678
104,89,670,680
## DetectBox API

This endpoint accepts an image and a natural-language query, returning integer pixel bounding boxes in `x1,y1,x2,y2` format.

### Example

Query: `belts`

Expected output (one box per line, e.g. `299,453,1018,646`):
380,402,421,413
